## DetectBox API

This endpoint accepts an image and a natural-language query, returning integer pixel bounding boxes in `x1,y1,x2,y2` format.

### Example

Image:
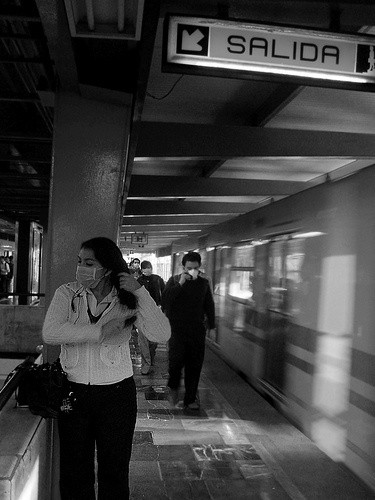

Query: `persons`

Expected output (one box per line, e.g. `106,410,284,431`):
42,236,171,500
135,261,165,374
0,255,13,298
128,259,142,280
162,253,215,410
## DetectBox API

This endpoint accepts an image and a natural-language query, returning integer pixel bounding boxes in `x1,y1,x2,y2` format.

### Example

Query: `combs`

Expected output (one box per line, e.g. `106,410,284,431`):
124,316,136,329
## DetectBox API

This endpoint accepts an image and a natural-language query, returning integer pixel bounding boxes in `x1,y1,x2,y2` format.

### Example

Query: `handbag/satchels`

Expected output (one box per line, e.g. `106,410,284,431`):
26,358,69,420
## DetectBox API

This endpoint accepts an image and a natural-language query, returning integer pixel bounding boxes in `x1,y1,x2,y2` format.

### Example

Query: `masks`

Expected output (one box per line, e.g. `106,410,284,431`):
141,269,152,276
187,269,199,280
133,263,140,268
76,266,106,288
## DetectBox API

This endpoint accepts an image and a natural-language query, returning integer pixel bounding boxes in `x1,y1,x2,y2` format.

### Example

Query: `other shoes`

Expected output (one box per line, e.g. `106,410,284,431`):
141,363,156,375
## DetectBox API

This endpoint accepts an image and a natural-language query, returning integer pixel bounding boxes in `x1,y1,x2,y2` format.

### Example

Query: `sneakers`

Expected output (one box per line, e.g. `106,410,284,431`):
187,403,200,410
168,389,178,406
